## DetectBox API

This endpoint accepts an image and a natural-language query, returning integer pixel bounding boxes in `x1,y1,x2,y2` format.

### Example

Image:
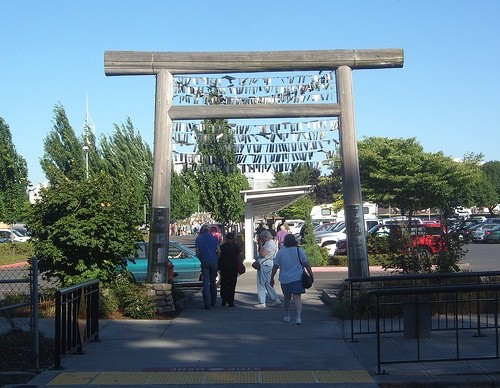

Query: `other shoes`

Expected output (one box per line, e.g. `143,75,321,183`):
210,304,215,307
229,303,235,307
205,305,210,309
222,300,227,305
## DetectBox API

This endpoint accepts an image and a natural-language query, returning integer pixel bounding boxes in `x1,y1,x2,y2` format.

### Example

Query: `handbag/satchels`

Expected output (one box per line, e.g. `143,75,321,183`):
235,261,246,275
252,262,261,270
303,271,312,289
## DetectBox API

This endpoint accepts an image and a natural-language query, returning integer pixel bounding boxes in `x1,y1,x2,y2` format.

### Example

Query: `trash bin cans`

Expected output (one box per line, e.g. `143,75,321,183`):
402,296,433,339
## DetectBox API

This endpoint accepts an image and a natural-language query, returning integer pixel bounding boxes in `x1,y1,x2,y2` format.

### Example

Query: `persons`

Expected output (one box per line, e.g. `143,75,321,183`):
256,219,289,249
254,230,281,307
270,234,313,323
172,222,202,235
195,225,221,309
218,233,241,306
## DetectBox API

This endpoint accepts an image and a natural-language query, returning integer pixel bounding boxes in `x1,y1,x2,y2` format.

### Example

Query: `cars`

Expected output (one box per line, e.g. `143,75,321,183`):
211,224,223,241
113,242,222,288
0,228,32,243
290,212,500,271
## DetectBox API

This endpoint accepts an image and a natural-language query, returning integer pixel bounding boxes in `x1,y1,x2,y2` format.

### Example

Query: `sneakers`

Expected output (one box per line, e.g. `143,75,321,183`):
270,300,281,307
294,318,302,324
283,316,291,322
254,304,267,308
